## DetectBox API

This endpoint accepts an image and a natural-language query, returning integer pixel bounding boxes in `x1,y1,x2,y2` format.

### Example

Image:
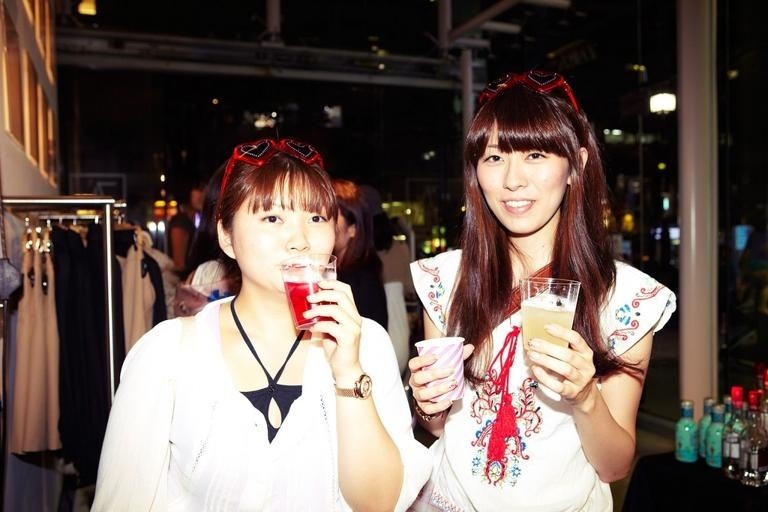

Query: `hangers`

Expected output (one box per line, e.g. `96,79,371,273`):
22,206,141,254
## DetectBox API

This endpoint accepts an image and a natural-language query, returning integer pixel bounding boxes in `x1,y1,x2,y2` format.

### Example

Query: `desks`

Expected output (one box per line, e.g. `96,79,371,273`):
624,450,768,512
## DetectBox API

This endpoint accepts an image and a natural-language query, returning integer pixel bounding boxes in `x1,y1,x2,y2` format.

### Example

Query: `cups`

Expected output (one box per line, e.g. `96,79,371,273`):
280,251,339,332
413,335,468,401
519,275,580,358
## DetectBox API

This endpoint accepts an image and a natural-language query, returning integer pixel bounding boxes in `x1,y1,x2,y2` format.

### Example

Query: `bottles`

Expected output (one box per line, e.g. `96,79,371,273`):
675,362,768,489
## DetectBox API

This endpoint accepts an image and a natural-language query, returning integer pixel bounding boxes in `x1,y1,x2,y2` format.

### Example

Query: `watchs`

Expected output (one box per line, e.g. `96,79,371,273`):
334,374,372,399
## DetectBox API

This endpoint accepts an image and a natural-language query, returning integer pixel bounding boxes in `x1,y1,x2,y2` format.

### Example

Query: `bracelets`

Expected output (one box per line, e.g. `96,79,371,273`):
412,398,443,423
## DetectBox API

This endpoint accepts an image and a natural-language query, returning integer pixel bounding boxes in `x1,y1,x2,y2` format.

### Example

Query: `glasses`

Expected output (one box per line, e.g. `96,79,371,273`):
212,135,326,229
479,67,582,128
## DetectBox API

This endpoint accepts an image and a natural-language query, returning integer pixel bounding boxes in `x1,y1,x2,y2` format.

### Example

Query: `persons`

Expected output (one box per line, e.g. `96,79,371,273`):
408,68,679,512
89,135,434,512
